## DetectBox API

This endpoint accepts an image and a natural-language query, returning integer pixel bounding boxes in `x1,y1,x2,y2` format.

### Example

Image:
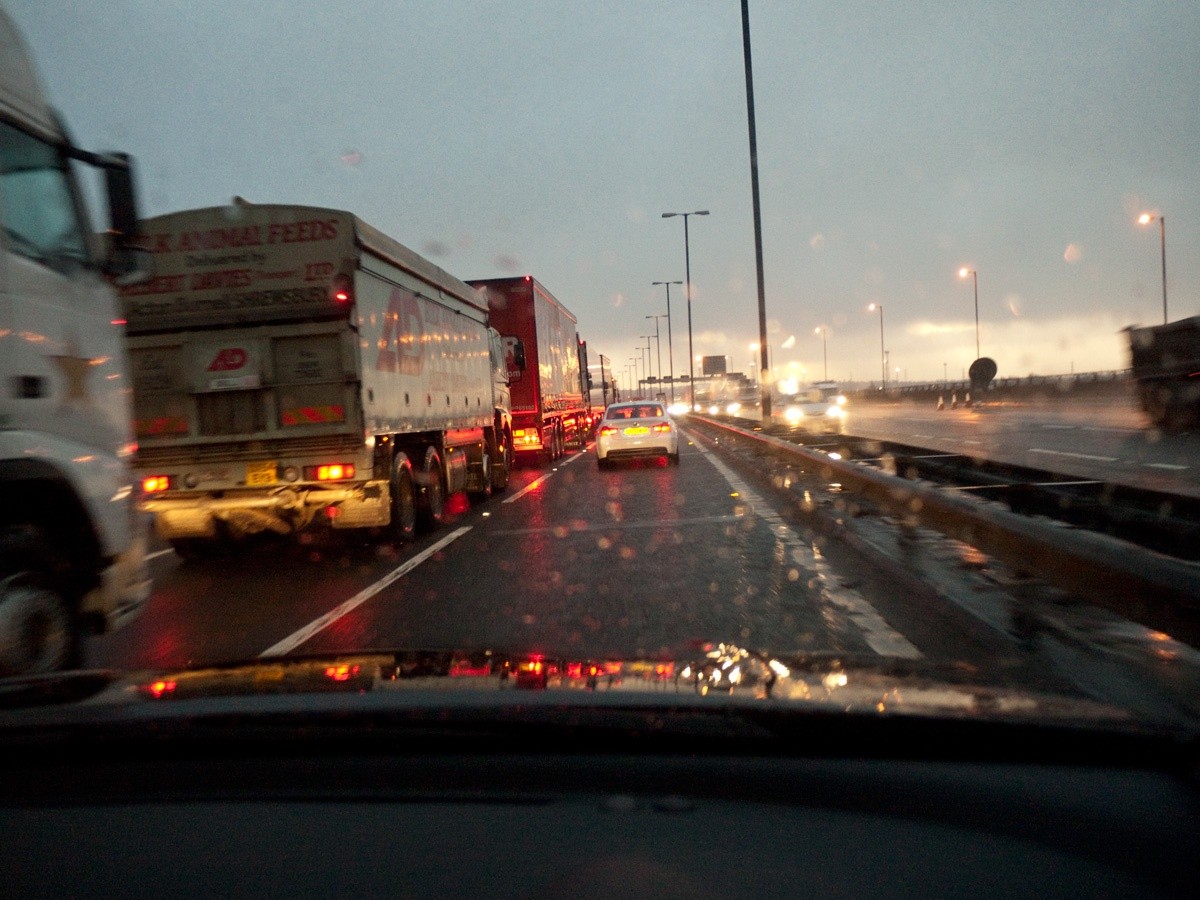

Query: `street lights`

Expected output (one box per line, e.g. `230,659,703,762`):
959,268,981,358
815,327,829,382
661,209,710,410
868,304,887,395
647,315,668,394
1139,213,1170,325
750,343,775,380
618,335,657,399
651,281,684,405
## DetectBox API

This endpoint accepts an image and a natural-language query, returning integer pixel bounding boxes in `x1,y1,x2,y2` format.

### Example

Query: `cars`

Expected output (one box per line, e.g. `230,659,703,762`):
784,391,844,434
592,399,681,467
710,399,736,415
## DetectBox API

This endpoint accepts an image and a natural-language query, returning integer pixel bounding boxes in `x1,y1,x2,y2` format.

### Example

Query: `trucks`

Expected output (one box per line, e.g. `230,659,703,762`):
0,7,152,702
738,386,759,407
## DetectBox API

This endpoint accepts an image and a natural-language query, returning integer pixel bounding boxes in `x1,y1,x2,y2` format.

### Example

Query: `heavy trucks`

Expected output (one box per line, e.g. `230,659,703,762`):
465,277,616,466
120,199,513,565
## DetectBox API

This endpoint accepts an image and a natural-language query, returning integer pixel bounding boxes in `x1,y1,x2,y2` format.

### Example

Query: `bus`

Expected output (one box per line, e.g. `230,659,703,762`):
808,384,838,401
